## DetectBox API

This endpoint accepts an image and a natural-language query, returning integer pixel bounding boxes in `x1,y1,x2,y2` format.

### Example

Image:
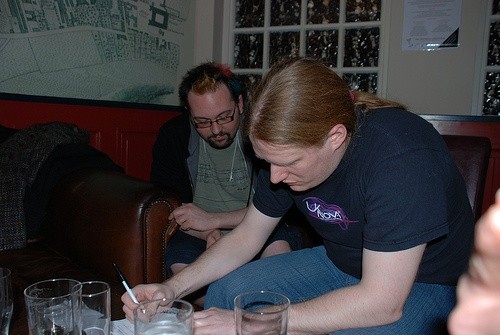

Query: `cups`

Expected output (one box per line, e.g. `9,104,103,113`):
71,281,111,335
0,268,15,335
134,298,194,335
233,290,291,335
24,278,83,335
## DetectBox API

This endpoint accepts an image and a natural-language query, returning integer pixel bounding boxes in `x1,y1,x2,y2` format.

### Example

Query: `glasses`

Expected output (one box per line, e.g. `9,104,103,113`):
187,103,237,128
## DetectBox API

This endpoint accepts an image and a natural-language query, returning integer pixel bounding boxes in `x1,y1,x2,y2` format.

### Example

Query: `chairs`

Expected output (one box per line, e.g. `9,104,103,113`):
0,121,205,335
439,135,492,225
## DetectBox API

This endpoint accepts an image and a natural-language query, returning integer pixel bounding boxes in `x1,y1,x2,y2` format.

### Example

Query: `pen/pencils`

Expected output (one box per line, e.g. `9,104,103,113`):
113,262,150,319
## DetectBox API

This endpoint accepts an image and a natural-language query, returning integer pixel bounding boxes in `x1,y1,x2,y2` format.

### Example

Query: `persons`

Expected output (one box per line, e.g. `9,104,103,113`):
149,61,309,308
122,56,477,335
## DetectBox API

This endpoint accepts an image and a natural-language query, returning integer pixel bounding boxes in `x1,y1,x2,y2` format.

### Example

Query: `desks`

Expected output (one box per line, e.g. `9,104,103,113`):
0,278,210,335
